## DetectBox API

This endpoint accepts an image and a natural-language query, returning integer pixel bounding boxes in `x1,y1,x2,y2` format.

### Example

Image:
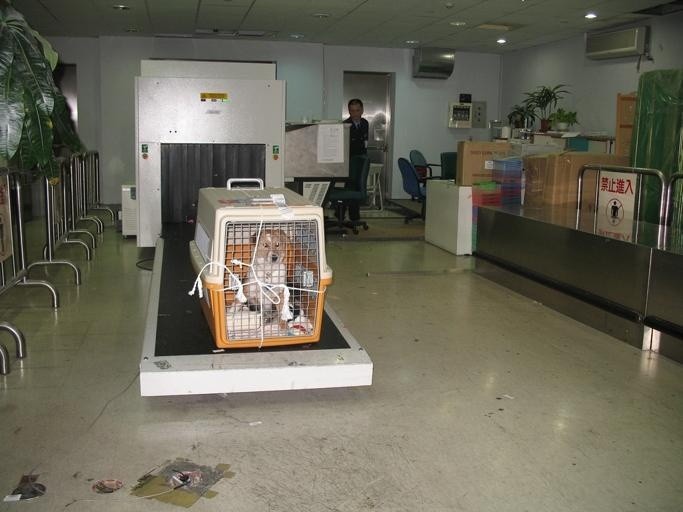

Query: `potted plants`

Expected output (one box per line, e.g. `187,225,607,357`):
505,83,582,132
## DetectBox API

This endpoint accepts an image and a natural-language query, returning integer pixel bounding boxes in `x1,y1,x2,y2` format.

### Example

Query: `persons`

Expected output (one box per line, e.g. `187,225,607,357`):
334,99,368,227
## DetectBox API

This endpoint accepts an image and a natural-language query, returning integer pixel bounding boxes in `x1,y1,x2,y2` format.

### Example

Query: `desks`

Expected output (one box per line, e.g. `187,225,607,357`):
424,178,473,257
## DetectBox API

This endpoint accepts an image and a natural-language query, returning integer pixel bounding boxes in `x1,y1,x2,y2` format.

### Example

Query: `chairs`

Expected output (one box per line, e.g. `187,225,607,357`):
398,149,441,218
322,155,370,238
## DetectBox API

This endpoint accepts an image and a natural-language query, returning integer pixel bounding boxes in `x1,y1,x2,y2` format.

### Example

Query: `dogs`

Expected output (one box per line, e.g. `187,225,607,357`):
229,229,295,330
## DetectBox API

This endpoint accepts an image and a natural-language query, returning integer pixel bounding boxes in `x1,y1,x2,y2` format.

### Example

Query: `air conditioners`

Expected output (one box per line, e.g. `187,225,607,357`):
583,25,646,58
